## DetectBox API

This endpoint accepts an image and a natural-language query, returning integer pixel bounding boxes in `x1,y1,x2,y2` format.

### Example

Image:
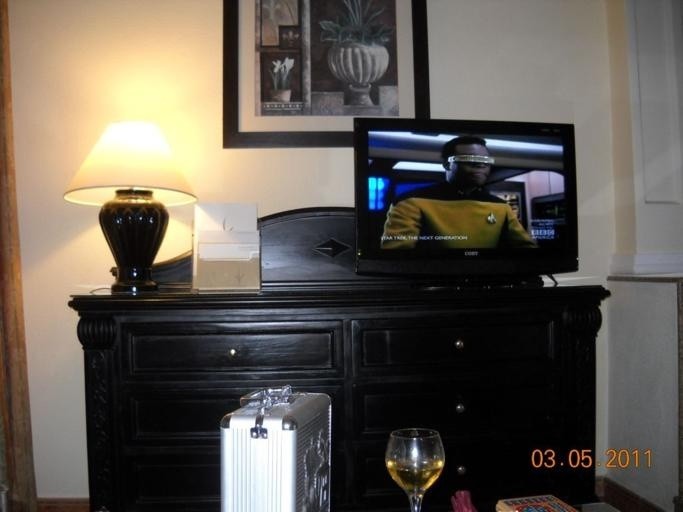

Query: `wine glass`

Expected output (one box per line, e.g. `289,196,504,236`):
384,428,445,512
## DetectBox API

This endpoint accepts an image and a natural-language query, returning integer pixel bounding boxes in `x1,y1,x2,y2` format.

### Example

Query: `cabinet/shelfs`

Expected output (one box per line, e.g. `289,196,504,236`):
65,284,613,510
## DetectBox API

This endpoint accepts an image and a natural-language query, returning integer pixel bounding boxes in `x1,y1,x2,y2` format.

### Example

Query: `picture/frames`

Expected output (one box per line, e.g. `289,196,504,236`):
220,1,431,152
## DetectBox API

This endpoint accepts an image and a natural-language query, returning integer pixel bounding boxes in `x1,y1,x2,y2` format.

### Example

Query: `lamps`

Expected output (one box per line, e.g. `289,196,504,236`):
61,120,199,295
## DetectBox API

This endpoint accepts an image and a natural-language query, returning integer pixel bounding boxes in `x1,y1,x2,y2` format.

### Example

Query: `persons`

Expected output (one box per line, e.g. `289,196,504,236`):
380,136,538,249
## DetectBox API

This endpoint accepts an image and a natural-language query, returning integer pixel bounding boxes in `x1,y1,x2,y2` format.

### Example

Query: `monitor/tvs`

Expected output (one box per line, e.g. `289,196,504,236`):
352,116,581,289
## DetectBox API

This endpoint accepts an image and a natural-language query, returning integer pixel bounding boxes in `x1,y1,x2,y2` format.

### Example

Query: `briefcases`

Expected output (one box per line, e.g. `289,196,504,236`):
217,385,333,512
